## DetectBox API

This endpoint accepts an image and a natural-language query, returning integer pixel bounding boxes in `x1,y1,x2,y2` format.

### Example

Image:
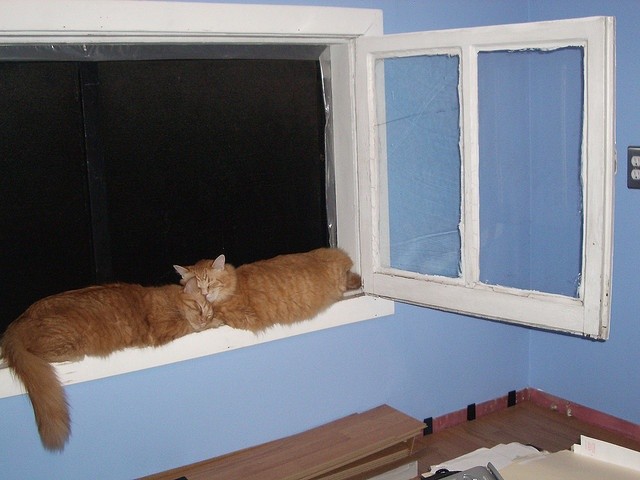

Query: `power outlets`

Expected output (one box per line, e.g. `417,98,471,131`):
626,146,640,189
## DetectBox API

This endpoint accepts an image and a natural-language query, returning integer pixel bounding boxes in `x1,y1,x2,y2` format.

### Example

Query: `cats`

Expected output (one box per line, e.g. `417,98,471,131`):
0,281,225,453
172,246,361,337
